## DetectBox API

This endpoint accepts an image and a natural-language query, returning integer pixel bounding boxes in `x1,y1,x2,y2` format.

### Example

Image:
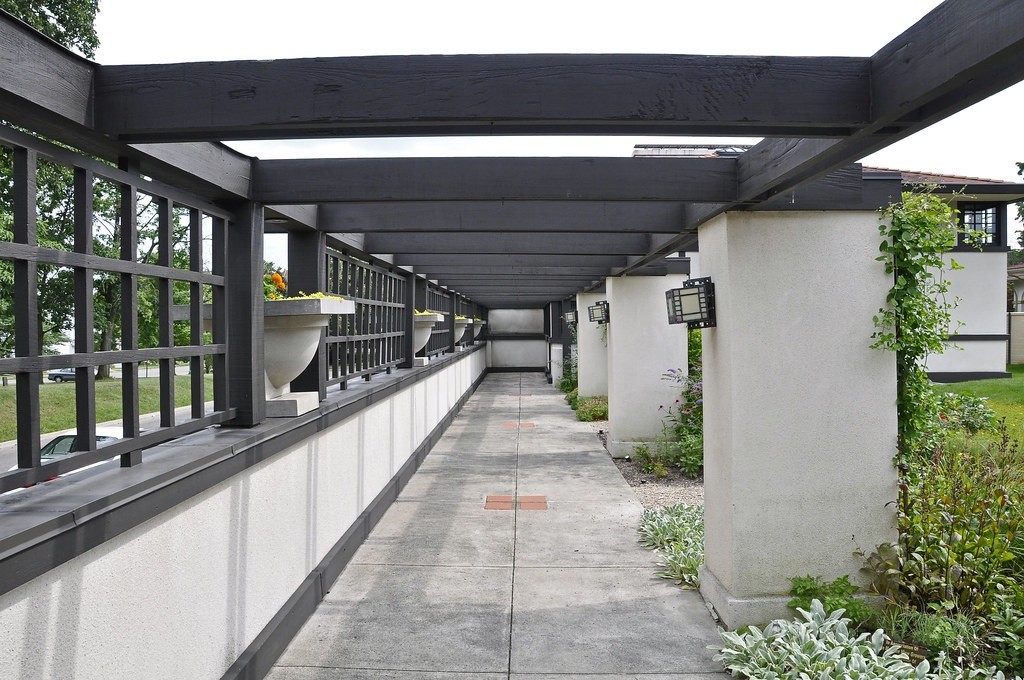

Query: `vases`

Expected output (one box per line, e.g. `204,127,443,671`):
173,299,355,418
474,320,488,344
455,319,473,352
414,315,445,367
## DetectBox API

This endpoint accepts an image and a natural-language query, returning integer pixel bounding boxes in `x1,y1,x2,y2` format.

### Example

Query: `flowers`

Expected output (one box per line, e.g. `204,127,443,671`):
455,314,467,319
262,271,344,301
415,309,441,315
473,318,481,321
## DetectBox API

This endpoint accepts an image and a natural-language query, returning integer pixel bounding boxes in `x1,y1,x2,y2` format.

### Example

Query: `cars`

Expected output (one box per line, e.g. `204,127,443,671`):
0,425,148,498
48,368,75,382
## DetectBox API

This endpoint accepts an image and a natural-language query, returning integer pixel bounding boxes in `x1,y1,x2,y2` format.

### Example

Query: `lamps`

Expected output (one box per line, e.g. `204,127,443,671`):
588,300,610,325
565,310,578,323
665,276,717,330
